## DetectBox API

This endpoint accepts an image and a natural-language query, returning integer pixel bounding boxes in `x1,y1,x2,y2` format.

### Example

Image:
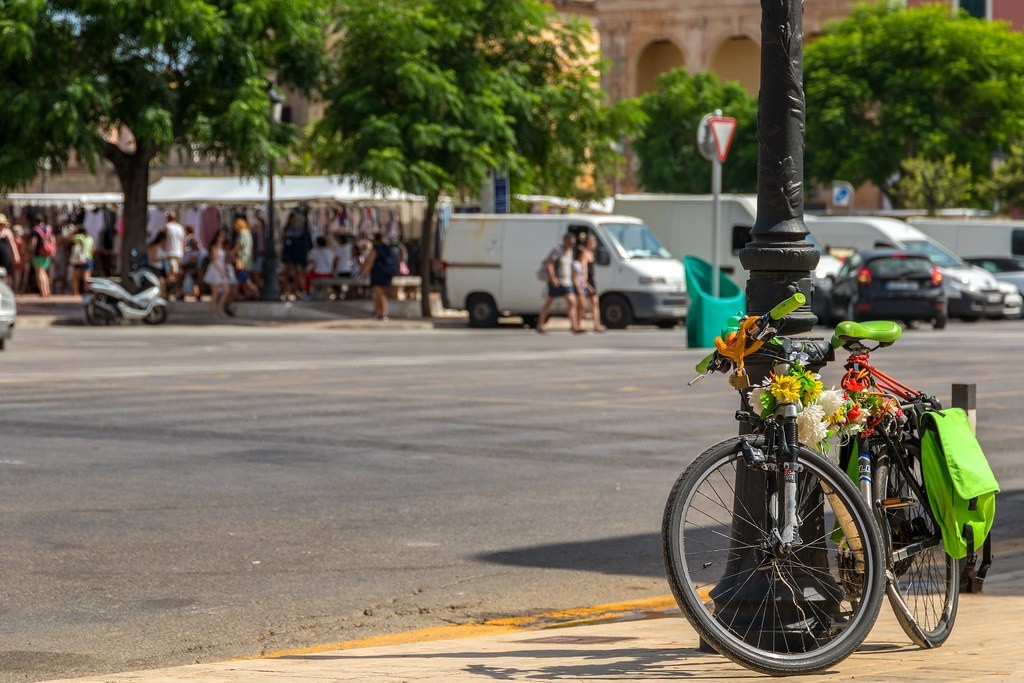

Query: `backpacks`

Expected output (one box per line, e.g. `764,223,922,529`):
34,224,55,257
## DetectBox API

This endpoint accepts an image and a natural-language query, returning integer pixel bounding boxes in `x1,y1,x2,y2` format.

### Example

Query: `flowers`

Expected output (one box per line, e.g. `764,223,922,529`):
746,344,883,455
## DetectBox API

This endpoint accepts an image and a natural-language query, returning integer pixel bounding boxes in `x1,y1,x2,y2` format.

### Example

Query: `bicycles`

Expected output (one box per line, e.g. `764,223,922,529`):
659,293,961,676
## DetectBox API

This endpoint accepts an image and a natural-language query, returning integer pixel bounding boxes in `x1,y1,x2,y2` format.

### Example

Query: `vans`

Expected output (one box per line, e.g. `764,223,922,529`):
614,194,763,274
440,213,690,330
809,214,1024,324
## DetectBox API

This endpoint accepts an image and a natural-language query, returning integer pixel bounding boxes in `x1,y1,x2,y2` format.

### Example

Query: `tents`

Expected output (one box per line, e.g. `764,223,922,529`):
147,172,456,256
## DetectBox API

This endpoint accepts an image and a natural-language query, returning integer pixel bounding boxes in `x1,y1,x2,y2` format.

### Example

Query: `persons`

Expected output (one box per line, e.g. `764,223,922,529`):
281,212,403,321
0,212,95,297
148,210,257,320
536,233,606,333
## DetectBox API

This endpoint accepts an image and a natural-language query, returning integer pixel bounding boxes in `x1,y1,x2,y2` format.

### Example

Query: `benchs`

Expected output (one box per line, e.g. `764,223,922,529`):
310,276,422,302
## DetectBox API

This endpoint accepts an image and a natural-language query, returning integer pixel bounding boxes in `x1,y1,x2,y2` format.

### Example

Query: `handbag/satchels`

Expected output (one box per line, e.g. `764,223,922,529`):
69,244,87,265
919,407,1001,560
830,437,859,550
538,248,560,281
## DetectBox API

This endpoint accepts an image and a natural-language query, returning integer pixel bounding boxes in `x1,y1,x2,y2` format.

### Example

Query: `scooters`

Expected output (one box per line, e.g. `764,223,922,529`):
82,247,169,327
